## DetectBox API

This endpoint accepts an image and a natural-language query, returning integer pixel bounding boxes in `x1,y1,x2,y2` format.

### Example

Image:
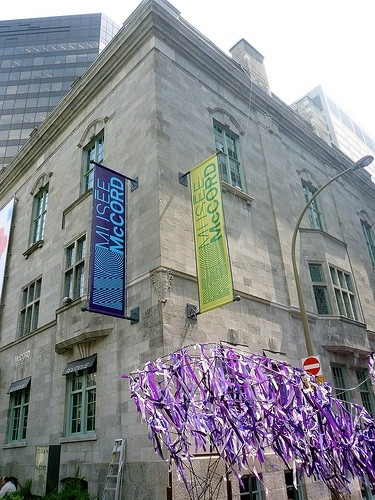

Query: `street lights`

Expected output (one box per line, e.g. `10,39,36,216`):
291,155,374,500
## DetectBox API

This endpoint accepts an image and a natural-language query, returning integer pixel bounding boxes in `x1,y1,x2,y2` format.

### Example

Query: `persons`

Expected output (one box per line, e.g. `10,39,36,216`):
0,477,16,499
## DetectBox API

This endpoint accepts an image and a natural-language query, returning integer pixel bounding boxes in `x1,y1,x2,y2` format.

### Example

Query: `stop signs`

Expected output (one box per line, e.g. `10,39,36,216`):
302,357,321,376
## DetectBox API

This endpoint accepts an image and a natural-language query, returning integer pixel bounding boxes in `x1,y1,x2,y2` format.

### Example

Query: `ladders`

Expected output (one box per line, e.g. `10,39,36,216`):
101,439,126,500
